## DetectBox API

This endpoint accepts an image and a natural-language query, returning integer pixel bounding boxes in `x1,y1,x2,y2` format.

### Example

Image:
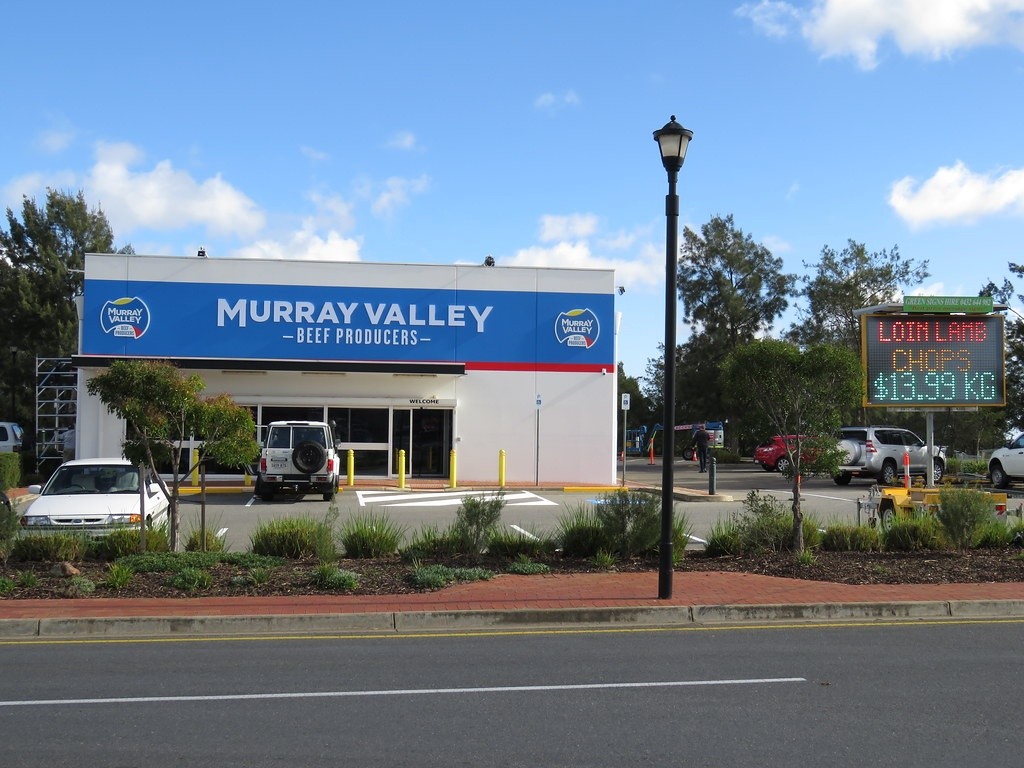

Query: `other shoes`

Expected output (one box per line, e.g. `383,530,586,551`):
699,470,707,473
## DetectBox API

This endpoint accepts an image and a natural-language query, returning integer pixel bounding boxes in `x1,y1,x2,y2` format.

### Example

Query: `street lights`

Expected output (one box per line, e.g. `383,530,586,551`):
650,116,696,598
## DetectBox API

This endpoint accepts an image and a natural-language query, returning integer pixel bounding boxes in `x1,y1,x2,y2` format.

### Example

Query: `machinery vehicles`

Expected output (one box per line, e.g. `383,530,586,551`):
626,424,705,456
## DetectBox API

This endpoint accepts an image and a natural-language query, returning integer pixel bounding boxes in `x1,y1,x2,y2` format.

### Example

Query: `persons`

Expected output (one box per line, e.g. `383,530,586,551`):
692,425,711,474
54,424,75,464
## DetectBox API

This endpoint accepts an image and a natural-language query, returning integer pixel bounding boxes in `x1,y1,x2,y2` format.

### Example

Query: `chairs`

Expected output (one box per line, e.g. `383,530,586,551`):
109,472,141,492
72,474,100,492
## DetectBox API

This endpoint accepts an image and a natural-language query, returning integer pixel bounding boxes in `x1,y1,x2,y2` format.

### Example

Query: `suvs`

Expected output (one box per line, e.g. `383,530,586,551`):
825,424,947,486
256,422,341,502
988,432,1024,490
0,423,25,455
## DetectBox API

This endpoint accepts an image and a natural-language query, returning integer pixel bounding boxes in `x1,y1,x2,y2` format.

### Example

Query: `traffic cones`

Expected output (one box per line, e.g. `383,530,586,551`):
620,451,624,462
692,451,701,462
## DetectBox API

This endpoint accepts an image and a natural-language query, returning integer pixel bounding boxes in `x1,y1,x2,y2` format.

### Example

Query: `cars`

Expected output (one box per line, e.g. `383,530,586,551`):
19,458,179,550
755,434,823,473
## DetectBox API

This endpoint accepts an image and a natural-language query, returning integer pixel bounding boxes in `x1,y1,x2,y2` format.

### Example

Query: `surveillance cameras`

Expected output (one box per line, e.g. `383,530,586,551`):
602,369,606,376
